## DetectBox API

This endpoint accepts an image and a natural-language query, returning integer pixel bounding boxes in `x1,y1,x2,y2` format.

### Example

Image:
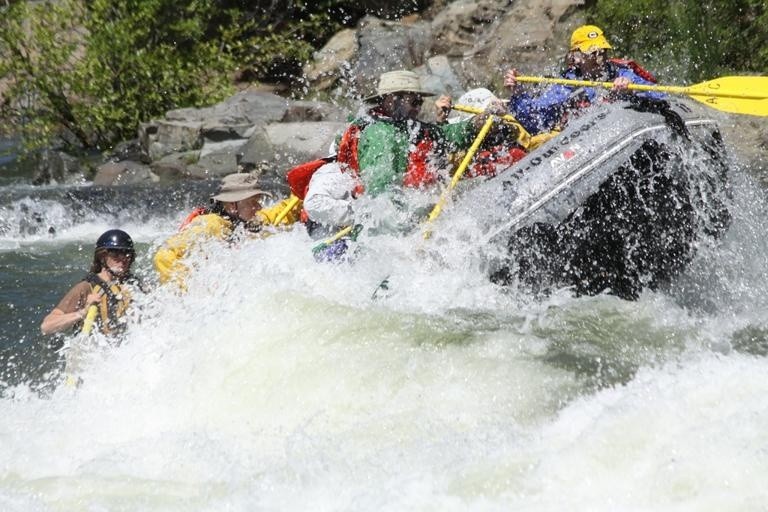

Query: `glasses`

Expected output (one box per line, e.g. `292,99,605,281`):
393,92,425,106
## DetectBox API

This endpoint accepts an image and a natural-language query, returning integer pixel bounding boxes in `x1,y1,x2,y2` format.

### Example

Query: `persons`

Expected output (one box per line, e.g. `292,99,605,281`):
39,229,147,384
357,68,492,239
152,172,303,297
282,115,384,262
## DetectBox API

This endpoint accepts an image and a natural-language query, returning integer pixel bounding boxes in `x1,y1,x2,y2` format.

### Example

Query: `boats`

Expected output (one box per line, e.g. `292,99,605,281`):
475,96,731,298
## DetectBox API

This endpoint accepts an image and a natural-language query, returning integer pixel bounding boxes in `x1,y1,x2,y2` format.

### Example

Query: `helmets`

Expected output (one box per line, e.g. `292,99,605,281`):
93,229,136,253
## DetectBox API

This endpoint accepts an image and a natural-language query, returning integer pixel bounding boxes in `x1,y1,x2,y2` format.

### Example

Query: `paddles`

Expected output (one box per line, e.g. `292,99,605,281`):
507,74,768,118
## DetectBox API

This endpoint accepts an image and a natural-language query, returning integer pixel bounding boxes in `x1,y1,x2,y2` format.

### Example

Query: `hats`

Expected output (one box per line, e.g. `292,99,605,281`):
455,87,512,124
209,171,274,204
361,69,438,106
569,24,613,56
